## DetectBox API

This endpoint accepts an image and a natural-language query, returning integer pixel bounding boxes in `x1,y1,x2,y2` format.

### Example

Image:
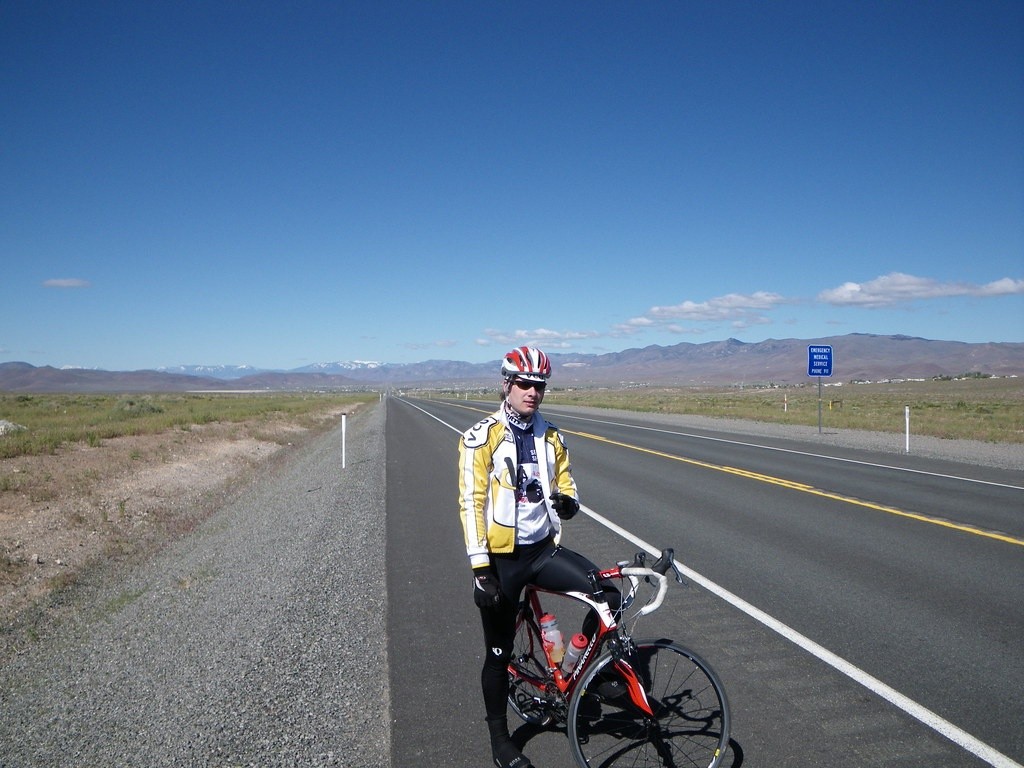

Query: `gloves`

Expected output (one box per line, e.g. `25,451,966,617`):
549,493,579,521
473,576,504,611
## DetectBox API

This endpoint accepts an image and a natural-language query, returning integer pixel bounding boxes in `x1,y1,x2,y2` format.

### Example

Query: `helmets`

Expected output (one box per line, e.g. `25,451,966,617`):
500,346,552,383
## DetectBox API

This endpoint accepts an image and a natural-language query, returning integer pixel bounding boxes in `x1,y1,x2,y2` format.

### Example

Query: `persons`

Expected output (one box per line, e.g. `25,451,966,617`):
457,346,624,768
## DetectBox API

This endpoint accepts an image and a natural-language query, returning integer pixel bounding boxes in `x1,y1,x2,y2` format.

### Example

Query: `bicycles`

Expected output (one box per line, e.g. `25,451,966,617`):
504,549,733,768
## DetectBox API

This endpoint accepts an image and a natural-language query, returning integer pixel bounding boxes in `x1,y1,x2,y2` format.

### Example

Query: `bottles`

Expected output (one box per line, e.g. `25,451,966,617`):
540,612,565,663
561,633,588,674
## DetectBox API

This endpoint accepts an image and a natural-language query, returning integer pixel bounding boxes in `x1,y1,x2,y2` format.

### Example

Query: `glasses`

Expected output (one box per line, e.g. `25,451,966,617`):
511,380,547,391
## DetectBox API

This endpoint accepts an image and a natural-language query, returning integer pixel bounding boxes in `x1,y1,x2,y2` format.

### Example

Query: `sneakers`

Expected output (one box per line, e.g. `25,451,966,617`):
485,714,530,768
595,672,627,700
